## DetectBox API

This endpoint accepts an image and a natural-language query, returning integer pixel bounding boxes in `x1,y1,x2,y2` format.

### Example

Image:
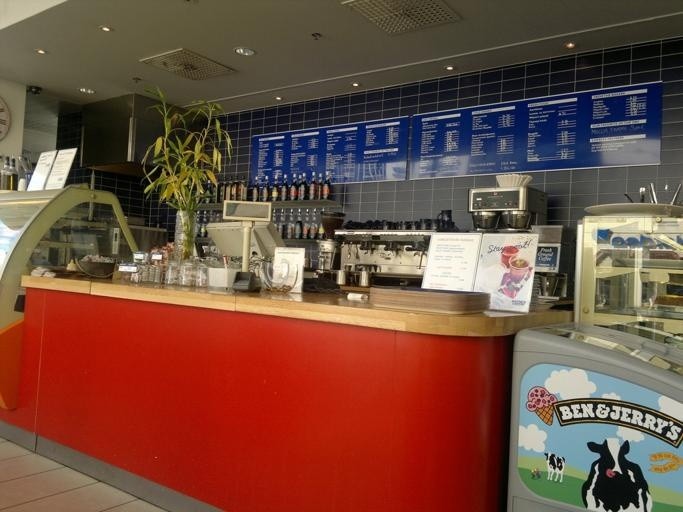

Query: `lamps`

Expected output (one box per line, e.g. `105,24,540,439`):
127,77,143,161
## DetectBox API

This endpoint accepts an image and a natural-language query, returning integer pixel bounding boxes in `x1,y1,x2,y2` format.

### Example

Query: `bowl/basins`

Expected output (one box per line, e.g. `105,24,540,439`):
77,260,114,279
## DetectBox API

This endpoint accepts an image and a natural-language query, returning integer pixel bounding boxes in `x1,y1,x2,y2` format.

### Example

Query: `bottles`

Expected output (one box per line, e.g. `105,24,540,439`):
271,176,280,200
287,209,295,239
260,176,270,201
205,180,213,203
215,209,223,222
195,211,201,236
309,172,316,200
317,207,327,239
207,209,214,222
280,174,289,201
230,176,237,200
323,171,331,199
290,174,298,200
301,173,308,200
316,173,323,200
310,209,318,239
302,209,310,239
225,181,232,200
201,211,207,236
218,182,225,202
295,209,302,238
7,159,18,191
278,208,287,239
252,177,260,202
272,209,277,229
298,175,303,200
237,175,247,201
1,157,10,189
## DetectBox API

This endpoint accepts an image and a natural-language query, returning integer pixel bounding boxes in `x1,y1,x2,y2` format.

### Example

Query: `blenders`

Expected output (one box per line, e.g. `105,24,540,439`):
315,210,346,283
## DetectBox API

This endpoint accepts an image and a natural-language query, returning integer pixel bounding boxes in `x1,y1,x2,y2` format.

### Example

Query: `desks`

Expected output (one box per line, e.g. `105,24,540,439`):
0,274,575,512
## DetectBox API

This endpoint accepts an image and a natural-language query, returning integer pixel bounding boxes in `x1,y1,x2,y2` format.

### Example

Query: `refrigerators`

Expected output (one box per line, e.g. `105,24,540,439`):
506,321,681,508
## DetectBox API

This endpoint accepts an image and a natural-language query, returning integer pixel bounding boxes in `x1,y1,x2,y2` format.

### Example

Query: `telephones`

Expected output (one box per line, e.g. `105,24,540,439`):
302,277,341,294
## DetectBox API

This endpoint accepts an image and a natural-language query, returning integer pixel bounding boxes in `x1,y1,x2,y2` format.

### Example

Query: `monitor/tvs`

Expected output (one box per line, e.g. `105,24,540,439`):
206,221,287,272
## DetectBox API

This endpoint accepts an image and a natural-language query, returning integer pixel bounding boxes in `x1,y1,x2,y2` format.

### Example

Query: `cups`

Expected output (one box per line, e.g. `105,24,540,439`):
164,261,179,285
437,208,452,220
112,263,162,286
179,262,194,285
343,219,456,232
194,263,209,287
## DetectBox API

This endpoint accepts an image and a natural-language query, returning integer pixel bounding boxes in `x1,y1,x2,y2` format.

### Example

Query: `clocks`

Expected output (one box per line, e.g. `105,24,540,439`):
0,96,11,141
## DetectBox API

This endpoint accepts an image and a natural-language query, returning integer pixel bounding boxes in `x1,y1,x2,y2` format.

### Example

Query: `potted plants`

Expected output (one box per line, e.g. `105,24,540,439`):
142,87,234,261
